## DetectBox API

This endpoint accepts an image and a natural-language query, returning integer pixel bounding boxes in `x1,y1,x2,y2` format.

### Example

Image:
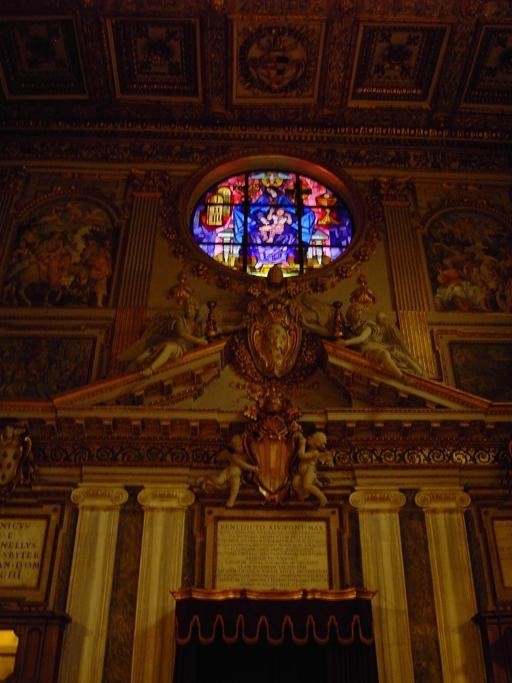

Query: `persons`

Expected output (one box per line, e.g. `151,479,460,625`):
291,429,333,511
4,197,112,307
332,304,436,382
249,186,300,269
429,211,510,312
204,434,260,510
132,297,210,377
258,206,293,242
